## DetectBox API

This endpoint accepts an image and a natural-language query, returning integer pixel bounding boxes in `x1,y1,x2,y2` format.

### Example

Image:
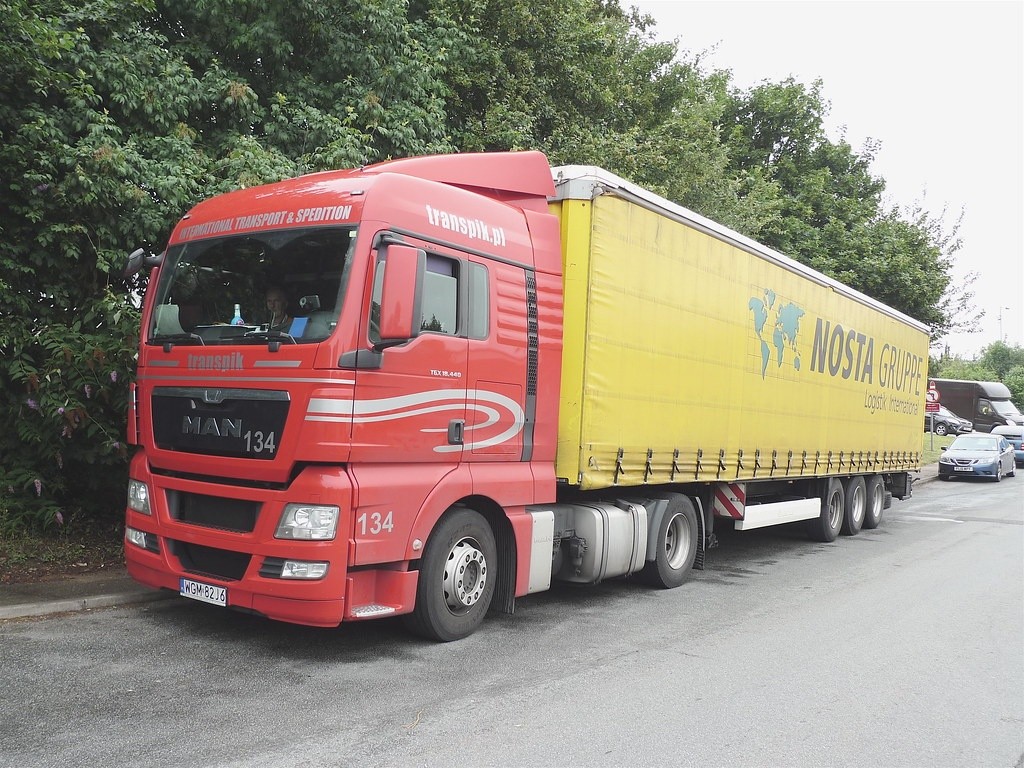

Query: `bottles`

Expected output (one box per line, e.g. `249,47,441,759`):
231,304,245,325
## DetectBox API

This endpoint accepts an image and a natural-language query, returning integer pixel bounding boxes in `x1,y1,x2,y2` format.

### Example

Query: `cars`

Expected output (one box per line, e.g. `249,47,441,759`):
924,402,973,437
990,425,1024,468
938,432,1017,482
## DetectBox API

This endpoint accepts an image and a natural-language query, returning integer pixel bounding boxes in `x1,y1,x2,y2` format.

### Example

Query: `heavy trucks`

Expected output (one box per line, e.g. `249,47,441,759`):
124,150,932,644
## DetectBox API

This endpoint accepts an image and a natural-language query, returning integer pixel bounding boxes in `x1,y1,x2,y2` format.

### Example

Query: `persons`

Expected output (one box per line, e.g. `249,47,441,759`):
212,285,293,328
983,403,992,415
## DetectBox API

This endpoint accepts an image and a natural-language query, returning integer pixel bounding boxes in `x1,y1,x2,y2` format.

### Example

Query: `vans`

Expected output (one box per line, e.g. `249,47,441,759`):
927,377,1024,434
